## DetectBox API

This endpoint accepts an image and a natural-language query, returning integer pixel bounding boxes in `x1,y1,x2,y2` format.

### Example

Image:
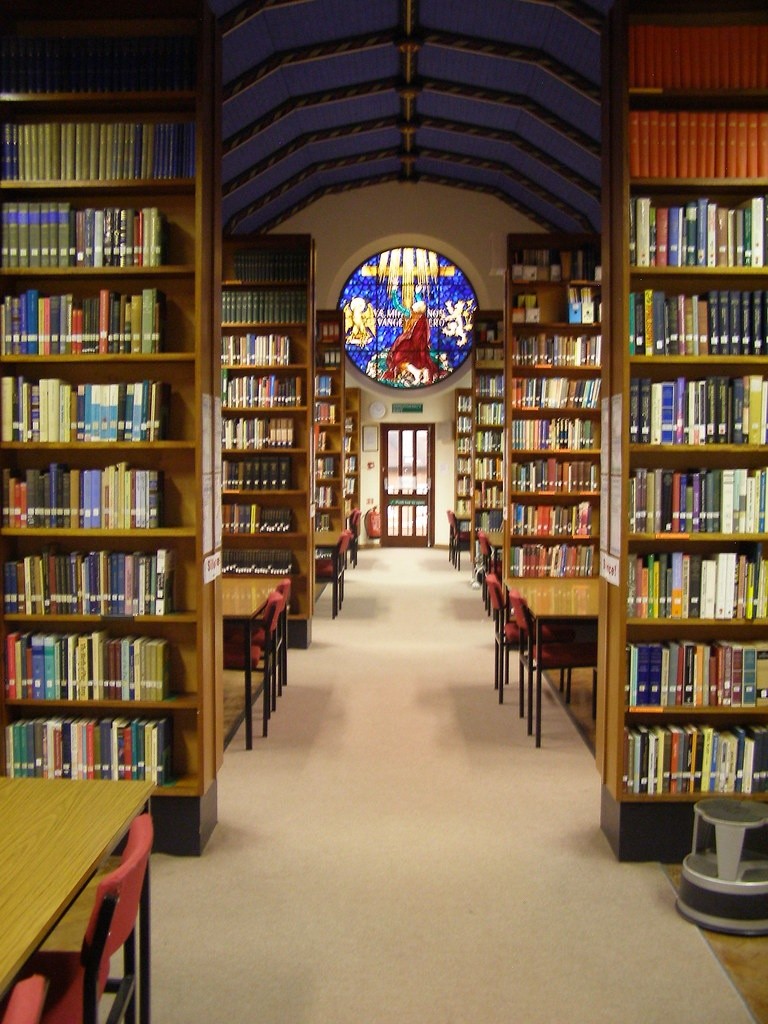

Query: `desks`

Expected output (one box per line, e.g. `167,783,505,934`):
223,579,291,750
316,532,341,620
487,533,503,574
456,513,471,570
0,778,157,1024
503,578,597,748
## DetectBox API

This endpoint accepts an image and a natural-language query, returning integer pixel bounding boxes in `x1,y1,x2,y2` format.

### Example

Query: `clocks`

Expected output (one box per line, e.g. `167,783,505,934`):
368,402,386,420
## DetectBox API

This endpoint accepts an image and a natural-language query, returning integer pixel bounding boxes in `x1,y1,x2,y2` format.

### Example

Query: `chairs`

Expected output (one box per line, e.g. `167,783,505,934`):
509,590,595,737
446,510,470,568
476,530,503,609
316,509,362,614
485,573,573,706
224,579,290,738
0,813,154,1024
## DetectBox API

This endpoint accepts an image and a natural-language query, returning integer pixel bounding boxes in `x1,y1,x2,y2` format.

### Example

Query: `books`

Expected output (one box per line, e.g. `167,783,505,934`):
456,23,768,802
0,36,357,788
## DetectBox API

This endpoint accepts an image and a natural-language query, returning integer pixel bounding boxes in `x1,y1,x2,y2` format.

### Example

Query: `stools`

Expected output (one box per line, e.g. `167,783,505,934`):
676,798,768,934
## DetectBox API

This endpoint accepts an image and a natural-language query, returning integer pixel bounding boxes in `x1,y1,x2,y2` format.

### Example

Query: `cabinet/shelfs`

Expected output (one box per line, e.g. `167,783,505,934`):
0,0,768,860
